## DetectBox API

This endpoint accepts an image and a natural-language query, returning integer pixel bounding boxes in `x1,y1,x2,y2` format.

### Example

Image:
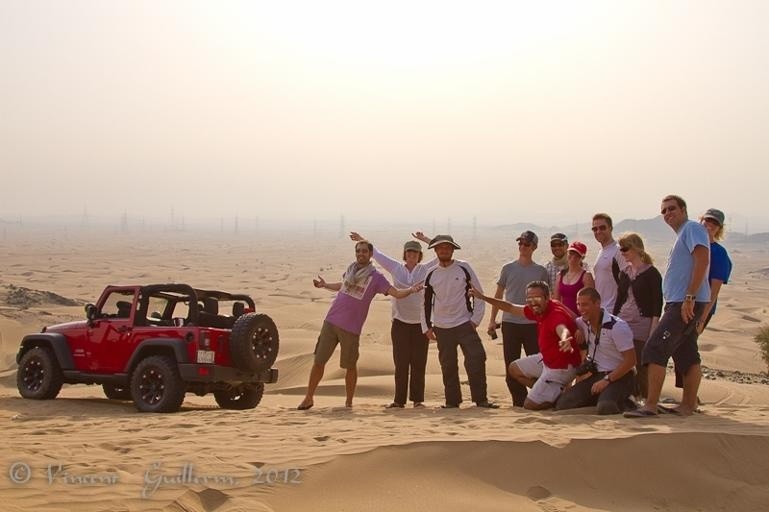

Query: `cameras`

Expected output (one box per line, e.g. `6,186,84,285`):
585,354,593,362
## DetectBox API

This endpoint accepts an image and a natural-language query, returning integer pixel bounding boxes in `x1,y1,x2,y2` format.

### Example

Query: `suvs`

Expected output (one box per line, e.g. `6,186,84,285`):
16,284,280,414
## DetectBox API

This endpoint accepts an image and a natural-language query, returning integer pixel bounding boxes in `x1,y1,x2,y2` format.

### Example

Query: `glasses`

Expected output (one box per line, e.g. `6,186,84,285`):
356,249,370,254
660,205,677,214
620,247,630,253
525,296,544,303
592,224,610,232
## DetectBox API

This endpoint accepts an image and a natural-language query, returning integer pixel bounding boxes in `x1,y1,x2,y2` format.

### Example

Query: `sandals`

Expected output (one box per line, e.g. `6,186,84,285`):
384,403,406,413
412,401,427,410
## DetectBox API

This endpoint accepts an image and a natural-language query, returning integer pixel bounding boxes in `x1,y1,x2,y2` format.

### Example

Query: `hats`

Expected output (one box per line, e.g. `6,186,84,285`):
403,241,422,253
427,234,462,249
703,207,724,228
516,229,538,248
567,240,588,257
551,232,568,243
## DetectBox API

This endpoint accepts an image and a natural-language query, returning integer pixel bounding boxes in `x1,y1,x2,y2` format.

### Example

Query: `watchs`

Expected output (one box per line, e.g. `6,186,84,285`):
682,295,696,302
604,375,612,385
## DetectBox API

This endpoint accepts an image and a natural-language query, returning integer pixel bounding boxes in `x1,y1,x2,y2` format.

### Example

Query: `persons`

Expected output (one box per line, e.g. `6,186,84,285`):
419,234,499,410
544,233,590,299
347,229,439,410
697,207,732,334
591,214,629,316
611,231,664,403
554,288,637,414
551,241,595,316
487,230,551,408
467,280,578,411
295,241,429,411
621,195,711,417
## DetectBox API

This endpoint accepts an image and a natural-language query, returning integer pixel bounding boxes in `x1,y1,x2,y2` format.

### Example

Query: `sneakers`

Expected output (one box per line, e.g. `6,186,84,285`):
440,404,459,409
477,399,500,409
623,407,658,418
657,403,678,416
